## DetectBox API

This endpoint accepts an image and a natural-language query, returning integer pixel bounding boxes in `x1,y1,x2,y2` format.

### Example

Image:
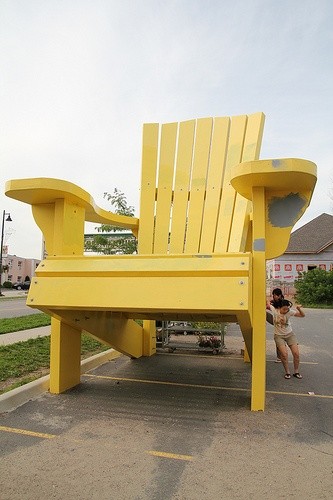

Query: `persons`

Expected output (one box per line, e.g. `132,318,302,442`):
266,288,291,363
265,299,305,378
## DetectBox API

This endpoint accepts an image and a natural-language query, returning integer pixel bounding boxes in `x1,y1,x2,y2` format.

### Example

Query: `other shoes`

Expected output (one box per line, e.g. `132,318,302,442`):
293,372,303,379
284,373,291,379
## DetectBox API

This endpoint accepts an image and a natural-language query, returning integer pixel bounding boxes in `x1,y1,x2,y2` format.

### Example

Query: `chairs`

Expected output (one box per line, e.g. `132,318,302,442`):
4,112,318,414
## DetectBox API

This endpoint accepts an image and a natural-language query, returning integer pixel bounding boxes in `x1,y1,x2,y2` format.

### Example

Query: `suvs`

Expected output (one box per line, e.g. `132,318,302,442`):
14,280,31,291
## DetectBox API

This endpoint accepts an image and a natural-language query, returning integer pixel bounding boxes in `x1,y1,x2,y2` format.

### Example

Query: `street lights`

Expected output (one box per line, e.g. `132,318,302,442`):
0,210,12,296
